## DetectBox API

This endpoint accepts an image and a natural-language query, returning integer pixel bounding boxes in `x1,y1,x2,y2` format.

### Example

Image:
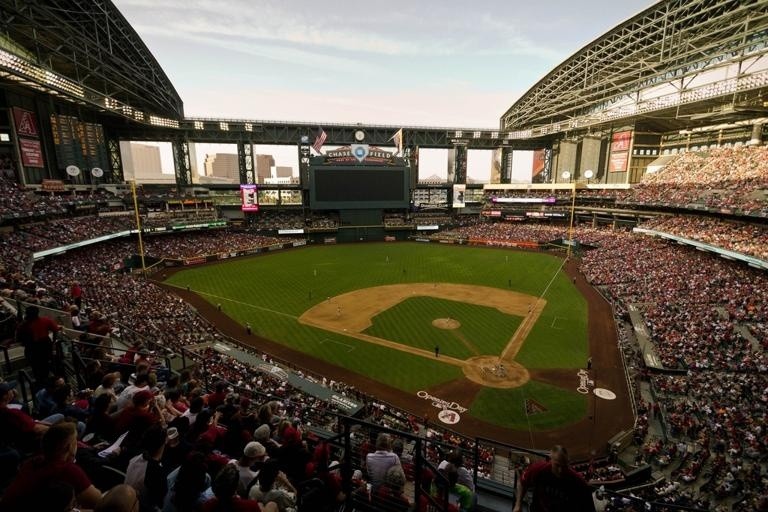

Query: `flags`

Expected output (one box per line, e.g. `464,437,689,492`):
310,126,327,153
393,129,403,152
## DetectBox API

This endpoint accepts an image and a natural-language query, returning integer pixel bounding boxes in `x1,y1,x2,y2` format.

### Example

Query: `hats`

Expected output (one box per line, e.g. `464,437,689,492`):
132,380,299,459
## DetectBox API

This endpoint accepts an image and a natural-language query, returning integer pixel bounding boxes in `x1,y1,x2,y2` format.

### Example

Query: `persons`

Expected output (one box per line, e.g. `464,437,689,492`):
0,146,768,512
